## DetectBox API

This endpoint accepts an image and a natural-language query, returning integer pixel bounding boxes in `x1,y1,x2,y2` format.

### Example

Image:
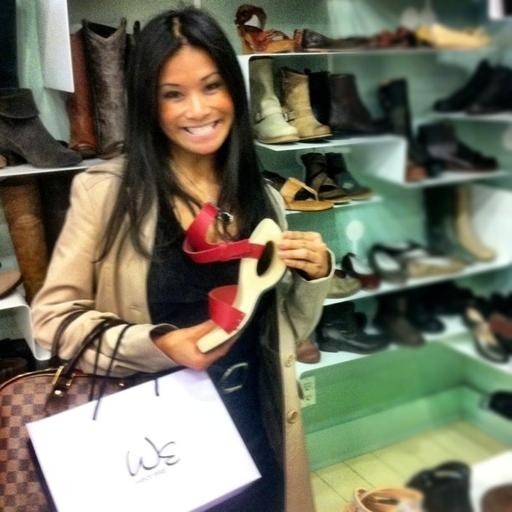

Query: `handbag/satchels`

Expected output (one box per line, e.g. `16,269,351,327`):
1,311,262,512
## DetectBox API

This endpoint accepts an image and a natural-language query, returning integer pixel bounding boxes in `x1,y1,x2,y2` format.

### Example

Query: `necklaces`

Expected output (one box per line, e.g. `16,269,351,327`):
178,171,234,225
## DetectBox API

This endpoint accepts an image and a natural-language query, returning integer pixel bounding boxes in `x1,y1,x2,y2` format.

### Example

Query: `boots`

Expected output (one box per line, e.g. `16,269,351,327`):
233,5,512,363
0,17,131,307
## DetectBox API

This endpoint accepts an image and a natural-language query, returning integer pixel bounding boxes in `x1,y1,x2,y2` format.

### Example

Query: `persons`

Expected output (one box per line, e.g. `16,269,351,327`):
27,7,336,511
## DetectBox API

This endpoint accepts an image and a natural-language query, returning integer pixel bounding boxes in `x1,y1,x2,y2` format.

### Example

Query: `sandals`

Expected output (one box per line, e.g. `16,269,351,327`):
340,461,512,512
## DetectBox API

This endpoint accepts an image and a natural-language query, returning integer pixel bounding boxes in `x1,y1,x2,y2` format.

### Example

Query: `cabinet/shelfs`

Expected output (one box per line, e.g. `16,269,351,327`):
238,45,512,378
0,156,131,363
